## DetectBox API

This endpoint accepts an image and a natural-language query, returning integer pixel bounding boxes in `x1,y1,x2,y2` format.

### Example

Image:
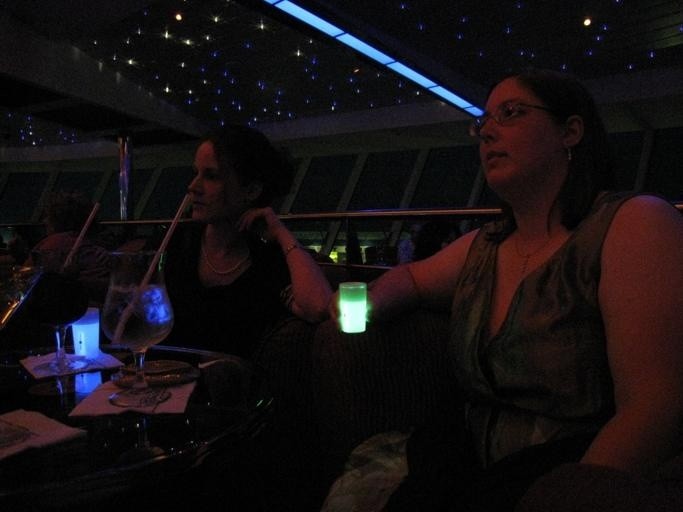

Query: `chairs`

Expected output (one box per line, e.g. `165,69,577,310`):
310,306,683,512
251,262,348,512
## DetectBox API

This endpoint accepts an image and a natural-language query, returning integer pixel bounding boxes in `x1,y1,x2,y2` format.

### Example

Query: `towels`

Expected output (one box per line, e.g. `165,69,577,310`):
0,408,88,462
19,352,125,380
69,379,198,417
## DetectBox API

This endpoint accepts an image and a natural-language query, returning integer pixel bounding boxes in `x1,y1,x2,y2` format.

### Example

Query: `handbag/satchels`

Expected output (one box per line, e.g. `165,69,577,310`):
319,428,419,512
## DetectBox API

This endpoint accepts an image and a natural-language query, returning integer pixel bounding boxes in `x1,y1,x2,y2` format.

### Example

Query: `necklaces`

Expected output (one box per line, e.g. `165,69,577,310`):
512,226,560,276
200,244,251,276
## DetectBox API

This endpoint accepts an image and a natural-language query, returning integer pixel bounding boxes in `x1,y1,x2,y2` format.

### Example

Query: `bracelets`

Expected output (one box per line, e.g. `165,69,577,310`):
283,242,299,256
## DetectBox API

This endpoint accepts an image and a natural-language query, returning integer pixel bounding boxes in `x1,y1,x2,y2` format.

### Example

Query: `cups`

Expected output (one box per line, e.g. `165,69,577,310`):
338,282,367,334
70,306,97,357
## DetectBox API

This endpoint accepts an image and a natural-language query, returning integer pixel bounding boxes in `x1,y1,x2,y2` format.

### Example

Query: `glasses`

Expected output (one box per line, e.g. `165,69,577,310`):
474,102,557,139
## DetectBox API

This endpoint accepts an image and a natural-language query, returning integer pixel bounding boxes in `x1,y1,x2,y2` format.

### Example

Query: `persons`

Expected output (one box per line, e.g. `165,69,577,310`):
22,189,113,286
328,65,683,510
417,221,461,258
156,123,336,373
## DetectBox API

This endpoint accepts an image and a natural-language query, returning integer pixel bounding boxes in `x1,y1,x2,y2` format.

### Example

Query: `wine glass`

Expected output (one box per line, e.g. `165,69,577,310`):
28,270,88,375
101,283,173,408
